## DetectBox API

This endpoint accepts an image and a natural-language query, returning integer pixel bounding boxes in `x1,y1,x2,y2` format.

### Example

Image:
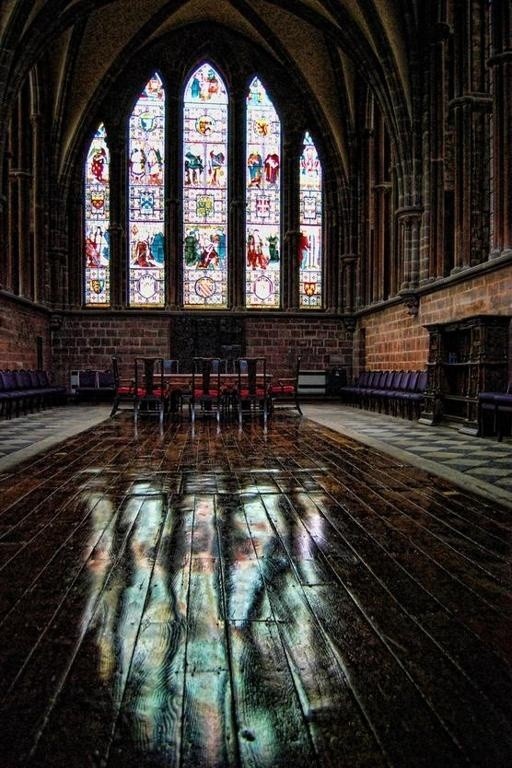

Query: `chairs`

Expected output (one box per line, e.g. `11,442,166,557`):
76,357,303,426
340,369,512,441
0,368,66,419
